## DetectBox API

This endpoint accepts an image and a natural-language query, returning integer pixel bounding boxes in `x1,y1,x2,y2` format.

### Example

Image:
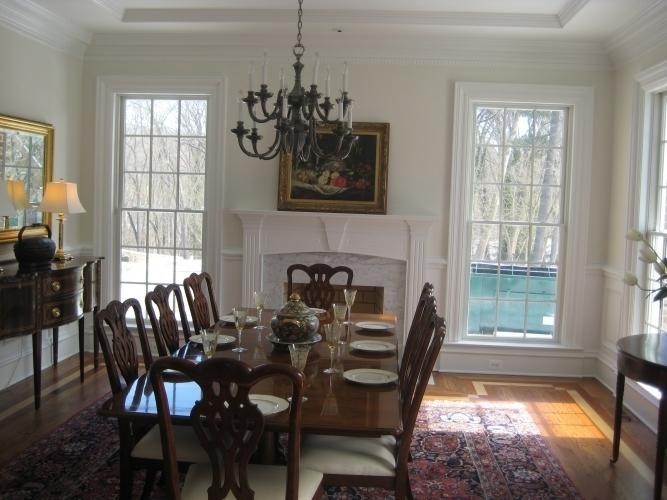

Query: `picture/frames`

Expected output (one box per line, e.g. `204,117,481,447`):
277,122,390,214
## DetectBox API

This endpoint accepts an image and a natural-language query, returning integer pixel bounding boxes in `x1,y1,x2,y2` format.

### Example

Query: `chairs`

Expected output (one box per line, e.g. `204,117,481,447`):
95,272,223,500
333,282,448,500
148,357,325,500
287,265,354,315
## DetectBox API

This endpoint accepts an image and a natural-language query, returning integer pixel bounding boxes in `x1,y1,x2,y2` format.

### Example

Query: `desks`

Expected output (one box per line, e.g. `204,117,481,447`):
609,331,667,500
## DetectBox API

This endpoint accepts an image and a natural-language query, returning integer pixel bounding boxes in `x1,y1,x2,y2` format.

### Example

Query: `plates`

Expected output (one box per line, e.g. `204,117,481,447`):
310,307,326,316
344,320,400,385
223,393,289,418
187,315,259,346
264,332,322,351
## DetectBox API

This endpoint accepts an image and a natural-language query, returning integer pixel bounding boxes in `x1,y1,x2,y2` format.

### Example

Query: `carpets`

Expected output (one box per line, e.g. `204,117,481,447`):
0,390,584,500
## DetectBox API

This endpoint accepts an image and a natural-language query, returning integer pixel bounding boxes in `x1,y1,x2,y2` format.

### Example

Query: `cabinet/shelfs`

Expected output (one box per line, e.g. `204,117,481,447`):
1,255,105,410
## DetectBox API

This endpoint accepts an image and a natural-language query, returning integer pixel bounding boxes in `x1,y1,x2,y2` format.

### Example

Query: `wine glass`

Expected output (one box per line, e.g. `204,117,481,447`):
323,287,359,377
199,291,268,365
286,342,312,405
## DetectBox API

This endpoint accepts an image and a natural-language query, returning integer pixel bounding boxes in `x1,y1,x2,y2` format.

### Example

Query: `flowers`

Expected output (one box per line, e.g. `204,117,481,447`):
621,228,667,308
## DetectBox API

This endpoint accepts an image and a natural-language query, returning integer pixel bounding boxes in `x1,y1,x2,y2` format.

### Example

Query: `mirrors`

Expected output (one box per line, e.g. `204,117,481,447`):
0,114,54,241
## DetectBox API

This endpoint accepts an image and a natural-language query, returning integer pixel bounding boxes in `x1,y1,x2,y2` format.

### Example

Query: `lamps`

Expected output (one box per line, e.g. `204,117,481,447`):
4,179,34,230
230,0,360,170
37,178,88,260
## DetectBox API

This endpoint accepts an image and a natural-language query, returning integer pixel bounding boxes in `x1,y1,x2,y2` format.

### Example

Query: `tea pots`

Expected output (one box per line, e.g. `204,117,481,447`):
271,294,320,342
